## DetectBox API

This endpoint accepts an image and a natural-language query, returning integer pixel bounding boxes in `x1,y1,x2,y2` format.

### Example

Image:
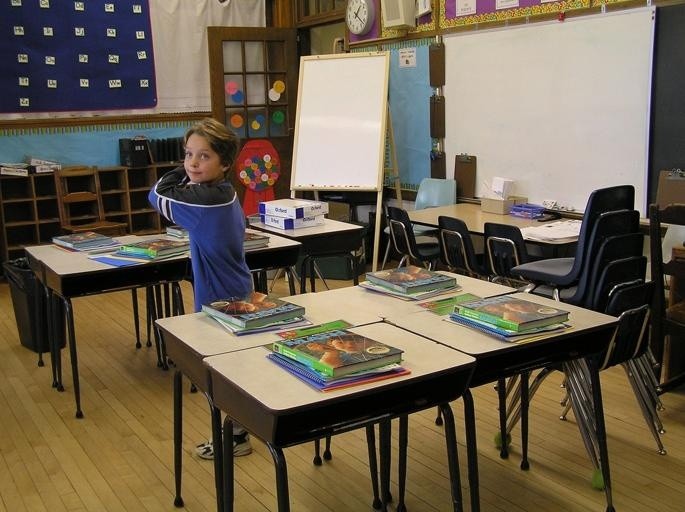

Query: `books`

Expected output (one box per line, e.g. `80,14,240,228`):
442,292,574,346
264,327,413,393
202,288,313,338
52,225,270,263
354,263,465,303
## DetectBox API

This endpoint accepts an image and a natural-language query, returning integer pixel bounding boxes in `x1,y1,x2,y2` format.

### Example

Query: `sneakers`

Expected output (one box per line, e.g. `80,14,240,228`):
196,431,252,458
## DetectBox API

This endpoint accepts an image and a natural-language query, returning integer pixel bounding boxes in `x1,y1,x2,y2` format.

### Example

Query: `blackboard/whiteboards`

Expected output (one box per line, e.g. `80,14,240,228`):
447,1,684,228
291,34,445,192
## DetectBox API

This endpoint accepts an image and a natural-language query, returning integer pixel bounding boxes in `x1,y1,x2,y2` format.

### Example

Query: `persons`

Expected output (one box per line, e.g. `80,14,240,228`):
148,116,255,460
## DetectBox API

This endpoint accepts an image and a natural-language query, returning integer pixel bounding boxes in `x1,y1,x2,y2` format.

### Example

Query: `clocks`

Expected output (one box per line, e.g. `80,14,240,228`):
345,0,375,36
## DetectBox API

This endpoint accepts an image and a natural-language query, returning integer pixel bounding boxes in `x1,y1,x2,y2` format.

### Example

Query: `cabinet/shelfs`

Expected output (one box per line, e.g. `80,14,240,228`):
0,158,185,283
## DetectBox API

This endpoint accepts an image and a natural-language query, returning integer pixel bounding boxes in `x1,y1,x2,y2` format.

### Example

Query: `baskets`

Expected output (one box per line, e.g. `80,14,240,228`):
509,203,545,220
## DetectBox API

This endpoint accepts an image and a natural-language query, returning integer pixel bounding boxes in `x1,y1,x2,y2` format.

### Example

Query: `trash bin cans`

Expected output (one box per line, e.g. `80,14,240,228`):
5,257,66,353
